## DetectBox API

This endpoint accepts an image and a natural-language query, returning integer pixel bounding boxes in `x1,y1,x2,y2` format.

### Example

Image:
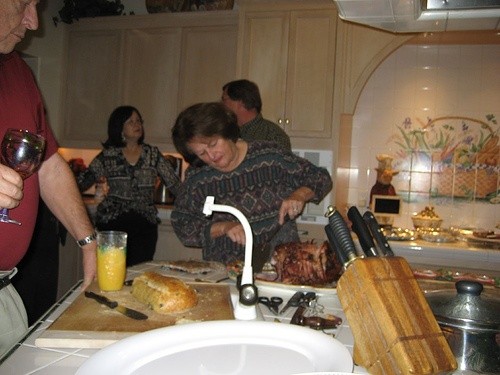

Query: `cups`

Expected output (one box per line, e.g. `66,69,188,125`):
96,231,127,291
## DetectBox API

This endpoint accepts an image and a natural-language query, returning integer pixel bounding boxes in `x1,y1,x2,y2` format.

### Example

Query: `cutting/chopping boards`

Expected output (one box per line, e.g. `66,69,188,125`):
36,278,235,350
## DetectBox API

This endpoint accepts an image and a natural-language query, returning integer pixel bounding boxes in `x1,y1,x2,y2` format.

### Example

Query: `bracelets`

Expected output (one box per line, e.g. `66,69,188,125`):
76,231,96,248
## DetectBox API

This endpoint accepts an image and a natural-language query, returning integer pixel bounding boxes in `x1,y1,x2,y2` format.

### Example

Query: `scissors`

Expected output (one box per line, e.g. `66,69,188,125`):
258,297,283,316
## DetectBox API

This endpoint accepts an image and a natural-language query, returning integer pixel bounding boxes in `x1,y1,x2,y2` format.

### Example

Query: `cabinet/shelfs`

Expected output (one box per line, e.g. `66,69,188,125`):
14,0,341,151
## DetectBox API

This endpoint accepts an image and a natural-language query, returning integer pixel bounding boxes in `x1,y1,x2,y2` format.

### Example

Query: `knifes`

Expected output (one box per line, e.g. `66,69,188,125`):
84,289,149,321
324,204,395,274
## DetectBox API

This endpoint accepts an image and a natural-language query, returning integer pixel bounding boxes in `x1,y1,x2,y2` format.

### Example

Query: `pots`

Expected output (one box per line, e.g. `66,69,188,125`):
423,279,500,375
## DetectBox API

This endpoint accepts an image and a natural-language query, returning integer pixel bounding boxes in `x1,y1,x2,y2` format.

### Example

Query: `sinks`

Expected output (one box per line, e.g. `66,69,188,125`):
73,319,355,375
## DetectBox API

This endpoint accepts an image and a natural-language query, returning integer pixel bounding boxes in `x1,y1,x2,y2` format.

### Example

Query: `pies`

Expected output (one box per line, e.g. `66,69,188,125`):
132,273,197,313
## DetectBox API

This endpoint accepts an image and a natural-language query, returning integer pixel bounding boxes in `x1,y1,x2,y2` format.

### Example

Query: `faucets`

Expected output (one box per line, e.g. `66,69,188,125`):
201,195,259,306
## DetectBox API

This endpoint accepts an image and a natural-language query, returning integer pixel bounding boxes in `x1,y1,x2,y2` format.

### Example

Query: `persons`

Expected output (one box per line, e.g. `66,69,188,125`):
0,0,96,363
77,105,180,265
171,102,333,268
221,79,291,150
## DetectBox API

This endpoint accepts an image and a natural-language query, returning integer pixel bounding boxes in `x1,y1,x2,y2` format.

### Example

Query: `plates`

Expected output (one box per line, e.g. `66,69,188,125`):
73,319,355,375
379,226,416,240
468,235,500,242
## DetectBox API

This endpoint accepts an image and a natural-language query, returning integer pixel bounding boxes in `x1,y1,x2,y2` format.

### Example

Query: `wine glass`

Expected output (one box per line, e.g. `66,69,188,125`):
0,128,45,225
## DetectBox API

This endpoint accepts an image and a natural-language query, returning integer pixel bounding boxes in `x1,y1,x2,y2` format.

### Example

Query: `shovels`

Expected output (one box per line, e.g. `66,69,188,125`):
252,216,290,274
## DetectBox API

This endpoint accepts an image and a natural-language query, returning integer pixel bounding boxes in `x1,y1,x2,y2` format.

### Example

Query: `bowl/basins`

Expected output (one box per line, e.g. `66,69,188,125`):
418,226,460,243
